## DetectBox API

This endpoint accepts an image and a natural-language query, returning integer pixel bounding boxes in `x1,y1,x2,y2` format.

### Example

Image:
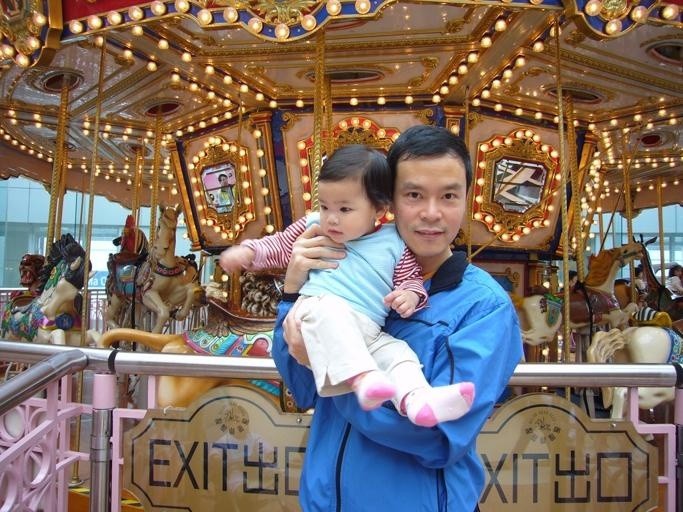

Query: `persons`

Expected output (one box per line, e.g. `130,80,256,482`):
559,270,577,293
214,173,236,213
665,264,683,295
218,144,474,427
119,215,147,255
271,125,524,512
635,267,649,295
